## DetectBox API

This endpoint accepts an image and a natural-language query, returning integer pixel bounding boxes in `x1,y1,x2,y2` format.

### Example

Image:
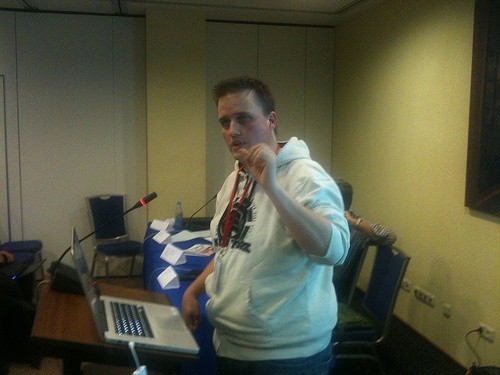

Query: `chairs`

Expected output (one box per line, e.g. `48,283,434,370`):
85,194,144,288
0,240,44,281
332,244,412,375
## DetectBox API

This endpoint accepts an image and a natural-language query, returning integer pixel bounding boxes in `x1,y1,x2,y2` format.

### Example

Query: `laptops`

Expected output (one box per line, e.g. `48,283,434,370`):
0,259,47,280
71,226,200,355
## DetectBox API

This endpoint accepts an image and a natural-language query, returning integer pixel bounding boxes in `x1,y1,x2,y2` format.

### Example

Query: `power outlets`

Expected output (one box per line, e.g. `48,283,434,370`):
401,278,495,342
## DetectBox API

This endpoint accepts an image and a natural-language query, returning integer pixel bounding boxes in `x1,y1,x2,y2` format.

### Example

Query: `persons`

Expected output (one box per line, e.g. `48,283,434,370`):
0,250,14,264
332,178,397,300
183,76,350,375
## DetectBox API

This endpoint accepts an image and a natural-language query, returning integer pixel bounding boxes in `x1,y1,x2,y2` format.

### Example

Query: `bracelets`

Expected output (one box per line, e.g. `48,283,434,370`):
355,219,362,226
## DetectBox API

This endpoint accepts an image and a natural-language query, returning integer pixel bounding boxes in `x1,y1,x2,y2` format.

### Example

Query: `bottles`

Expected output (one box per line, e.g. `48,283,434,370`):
175,200,184,228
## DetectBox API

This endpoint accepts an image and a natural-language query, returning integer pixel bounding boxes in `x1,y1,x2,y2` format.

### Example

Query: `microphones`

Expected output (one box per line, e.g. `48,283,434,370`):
48,192,157,295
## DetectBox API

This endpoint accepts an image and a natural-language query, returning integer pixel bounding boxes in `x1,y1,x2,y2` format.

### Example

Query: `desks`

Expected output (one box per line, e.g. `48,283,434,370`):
142,221,223,375
31,274,200,375
